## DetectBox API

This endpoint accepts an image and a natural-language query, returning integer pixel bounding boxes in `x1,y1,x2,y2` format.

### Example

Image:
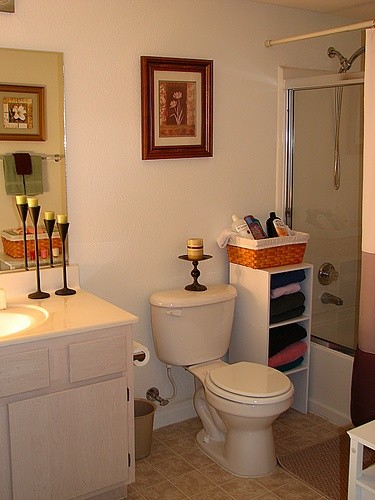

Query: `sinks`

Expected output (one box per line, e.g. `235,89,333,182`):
0,305,49,337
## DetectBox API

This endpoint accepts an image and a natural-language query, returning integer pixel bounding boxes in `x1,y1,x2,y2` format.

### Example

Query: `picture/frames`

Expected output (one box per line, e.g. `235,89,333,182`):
0,82,46,142
140,55,214,159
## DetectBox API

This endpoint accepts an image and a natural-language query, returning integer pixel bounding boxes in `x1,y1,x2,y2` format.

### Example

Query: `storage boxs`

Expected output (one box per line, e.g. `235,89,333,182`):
225,223,311,269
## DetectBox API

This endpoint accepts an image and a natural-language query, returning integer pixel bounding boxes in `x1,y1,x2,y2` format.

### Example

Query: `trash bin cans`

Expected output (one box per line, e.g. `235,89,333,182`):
133,397,157,461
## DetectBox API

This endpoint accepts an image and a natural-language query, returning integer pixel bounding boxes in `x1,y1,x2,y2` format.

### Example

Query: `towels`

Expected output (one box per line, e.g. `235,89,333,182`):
3,155,43,196
13,154,32,175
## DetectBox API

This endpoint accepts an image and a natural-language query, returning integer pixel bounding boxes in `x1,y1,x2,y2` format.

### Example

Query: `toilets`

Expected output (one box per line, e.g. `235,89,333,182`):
149,283,295,479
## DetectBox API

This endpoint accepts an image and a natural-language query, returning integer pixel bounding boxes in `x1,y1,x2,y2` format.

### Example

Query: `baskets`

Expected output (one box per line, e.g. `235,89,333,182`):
227,229,310,269
0,225,63,258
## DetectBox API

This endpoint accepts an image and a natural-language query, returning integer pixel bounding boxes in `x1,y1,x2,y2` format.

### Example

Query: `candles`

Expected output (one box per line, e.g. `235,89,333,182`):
43,212,55,220
57,215,69,225
187,238,204,258
27,199,38,207
15,196,27,206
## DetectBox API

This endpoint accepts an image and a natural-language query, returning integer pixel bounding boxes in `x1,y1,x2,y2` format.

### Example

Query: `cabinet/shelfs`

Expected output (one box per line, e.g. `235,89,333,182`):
229,262,314,414
0,265,139,499
346,420,375,500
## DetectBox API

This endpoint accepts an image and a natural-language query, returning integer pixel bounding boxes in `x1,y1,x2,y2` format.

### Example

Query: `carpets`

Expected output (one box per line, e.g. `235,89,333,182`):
276,426,375,500
